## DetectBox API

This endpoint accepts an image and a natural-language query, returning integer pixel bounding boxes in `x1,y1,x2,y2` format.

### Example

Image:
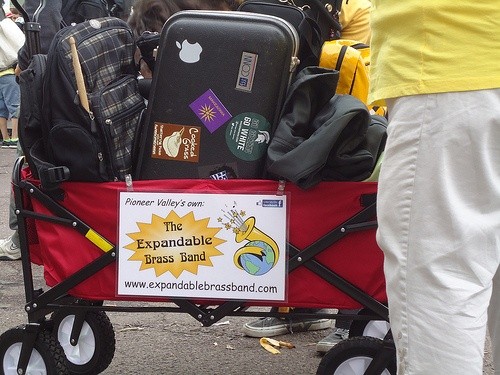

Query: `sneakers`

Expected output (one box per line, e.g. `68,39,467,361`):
9,139,18,148
316,328,350,352
2,138,10,148
243,311,331,336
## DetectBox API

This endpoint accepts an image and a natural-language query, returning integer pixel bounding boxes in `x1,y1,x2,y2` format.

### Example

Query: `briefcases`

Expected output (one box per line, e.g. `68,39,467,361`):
129,9,298,182
291,0,343,41
236,1,322,119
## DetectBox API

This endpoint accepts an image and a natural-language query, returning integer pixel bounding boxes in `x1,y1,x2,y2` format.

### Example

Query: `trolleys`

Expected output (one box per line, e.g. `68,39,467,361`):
0,152,397,375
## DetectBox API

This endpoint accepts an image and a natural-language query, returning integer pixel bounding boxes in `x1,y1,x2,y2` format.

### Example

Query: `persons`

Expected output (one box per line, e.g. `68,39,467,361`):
367,0,500,375
0,0,372,353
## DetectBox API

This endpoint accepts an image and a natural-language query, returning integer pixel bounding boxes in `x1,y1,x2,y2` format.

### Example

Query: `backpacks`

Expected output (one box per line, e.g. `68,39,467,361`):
36,122,108,183
32,14,145,183
